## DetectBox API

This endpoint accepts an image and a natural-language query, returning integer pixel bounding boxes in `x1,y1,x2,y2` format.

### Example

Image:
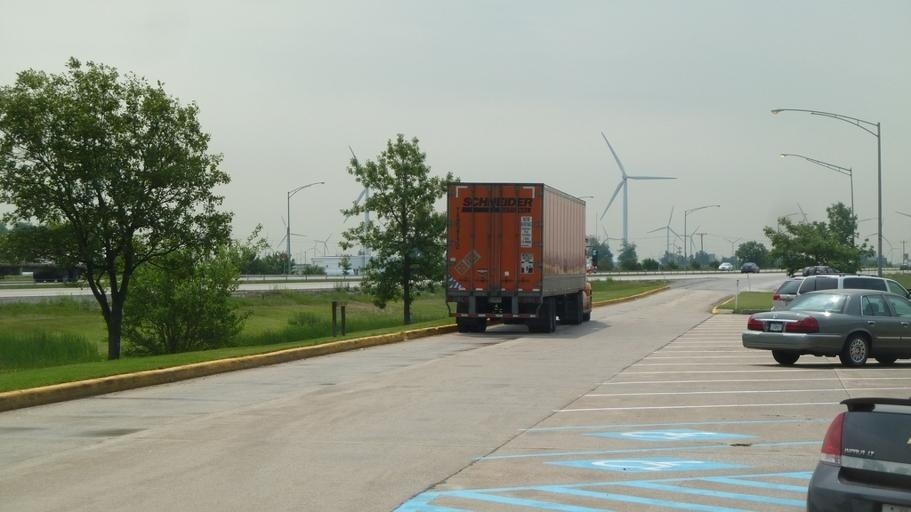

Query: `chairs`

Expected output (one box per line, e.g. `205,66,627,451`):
863,302,880,315
882,302,894,316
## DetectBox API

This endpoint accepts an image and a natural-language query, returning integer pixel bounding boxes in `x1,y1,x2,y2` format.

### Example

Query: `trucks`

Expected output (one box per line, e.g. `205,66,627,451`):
445,181,599,333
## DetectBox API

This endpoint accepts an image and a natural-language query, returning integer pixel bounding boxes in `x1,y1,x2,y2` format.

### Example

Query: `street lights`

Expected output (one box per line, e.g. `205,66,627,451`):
780,154,855,248
286,182,326,274
685,205,721,265
770,108,882,276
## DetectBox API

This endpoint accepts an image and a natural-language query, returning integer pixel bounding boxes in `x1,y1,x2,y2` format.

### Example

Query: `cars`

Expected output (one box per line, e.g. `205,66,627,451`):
807,395,911,511
741,262,761,273
719,262,734,271
743,265,911,365
